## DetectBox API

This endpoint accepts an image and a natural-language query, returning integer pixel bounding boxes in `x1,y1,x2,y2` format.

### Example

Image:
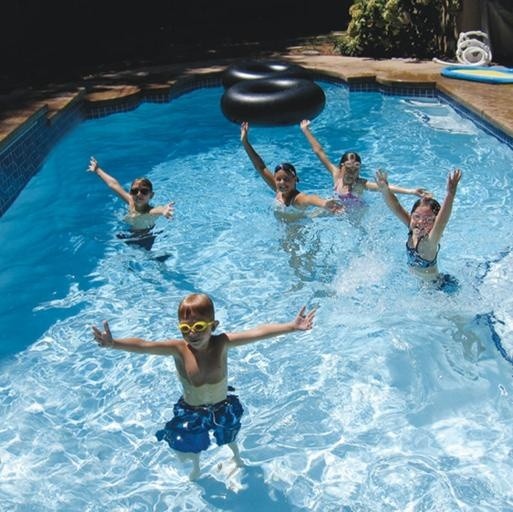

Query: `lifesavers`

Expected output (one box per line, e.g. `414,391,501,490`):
221,58,325,127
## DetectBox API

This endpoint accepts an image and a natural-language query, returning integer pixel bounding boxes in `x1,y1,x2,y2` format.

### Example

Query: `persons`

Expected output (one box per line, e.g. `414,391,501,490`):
91,293,317,482
240,122,346,270
87,157,176,251
372,167,463,293
300,119,433,226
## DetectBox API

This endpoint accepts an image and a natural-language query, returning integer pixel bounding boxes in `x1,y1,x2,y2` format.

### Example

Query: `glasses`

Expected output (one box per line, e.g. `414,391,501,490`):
178,320,215,334
275,164,296,177
130,188,151,195
412,213,434,224
341,161,360,168
